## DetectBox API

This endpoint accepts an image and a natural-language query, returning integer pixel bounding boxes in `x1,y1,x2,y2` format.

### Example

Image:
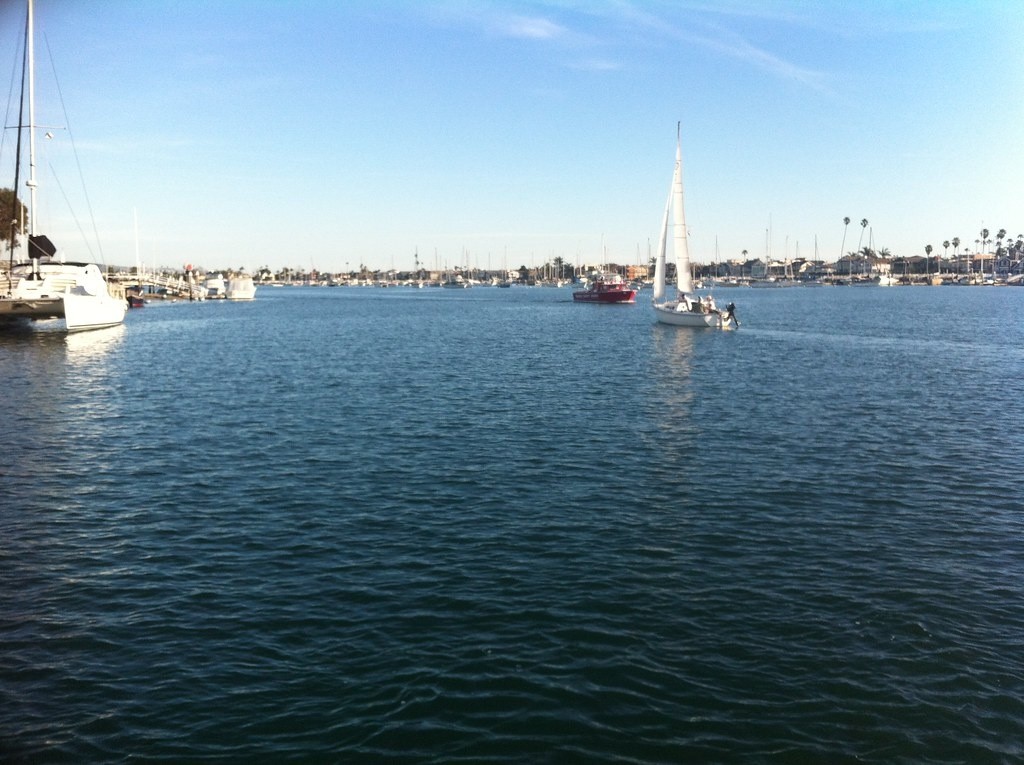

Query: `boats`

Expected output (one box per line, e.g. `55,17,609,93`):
572,271,640,304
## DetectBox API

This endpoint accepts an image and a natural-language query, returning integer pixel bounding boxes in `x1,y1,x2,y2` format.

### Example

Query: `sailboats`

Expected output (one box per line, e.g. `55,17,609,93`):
125,240,595,310
606,214,1024,291
0,1,126,337
651,121,740,331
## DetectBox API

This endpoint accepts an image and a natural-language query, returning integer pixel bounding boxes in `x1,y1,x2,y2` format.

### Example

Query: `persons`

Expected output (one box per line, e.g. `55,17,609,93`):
695,297,705,314
706,296,715,312
